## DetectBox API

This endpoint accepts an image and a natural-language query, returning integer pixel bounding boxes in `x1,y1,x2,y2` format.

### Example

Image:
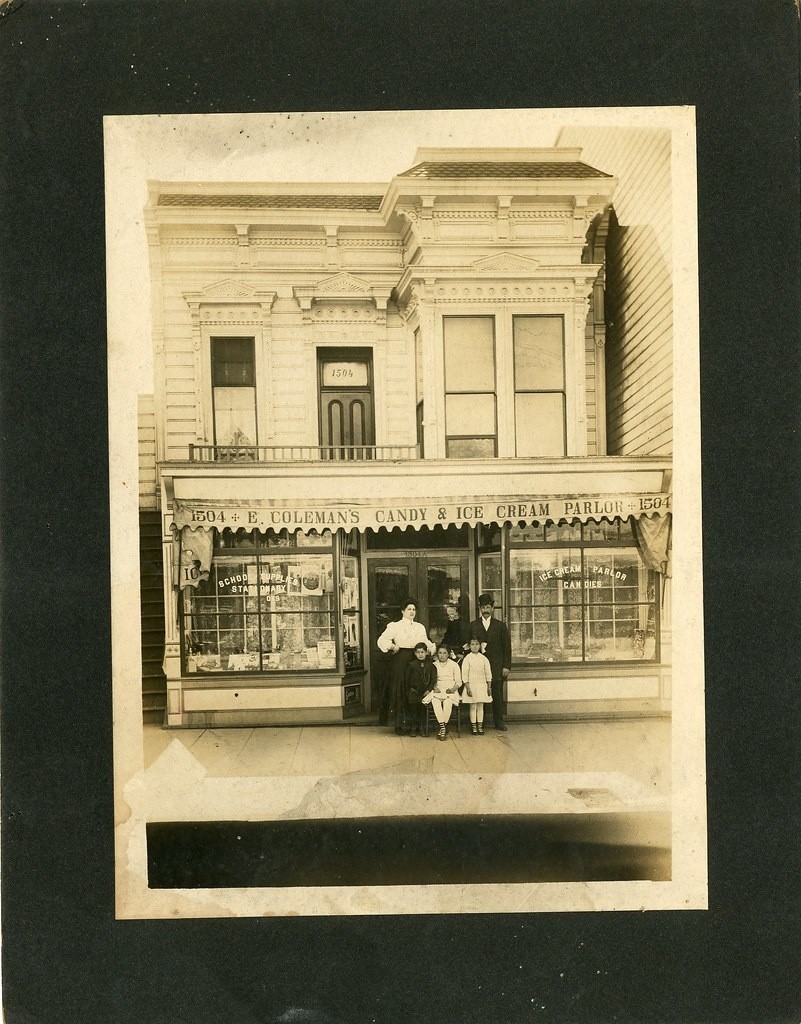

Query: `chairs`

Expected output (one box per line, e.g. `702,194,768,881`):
425,647,461,738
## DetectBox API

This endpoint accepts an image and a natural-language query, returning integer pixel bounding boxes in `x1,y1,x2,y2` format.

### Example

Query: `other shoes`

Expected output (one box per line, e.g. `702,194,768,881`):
494,721,507,730
395,726,404,735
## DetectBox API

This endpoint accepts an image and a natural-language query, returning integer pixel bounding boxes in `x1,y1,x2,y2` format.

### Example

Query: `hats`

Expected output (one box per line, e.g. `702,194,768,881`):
477,594,495,605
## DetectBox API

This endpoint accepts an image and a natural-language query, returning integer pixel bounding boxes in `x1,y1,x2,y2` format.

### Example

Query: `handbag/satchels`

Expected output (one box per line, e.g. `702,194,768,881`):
422,690,435,705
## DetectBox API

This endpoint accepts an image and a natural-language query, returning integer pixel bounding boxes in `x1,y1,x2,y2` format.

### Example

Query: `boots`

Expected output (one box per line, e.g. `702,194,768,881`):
477,722,484,735
420,722,427,736
409,721,417,736
471,723,478,735
437,722,447,740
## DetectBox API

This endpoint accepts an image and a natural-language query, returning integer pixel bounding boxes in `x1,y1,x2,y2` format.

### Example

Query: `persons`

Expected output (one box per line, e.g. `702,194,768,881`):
376,598,437,727
421,644,462,740
465,594,511,731
462,637,493,736
404,642,437,737
